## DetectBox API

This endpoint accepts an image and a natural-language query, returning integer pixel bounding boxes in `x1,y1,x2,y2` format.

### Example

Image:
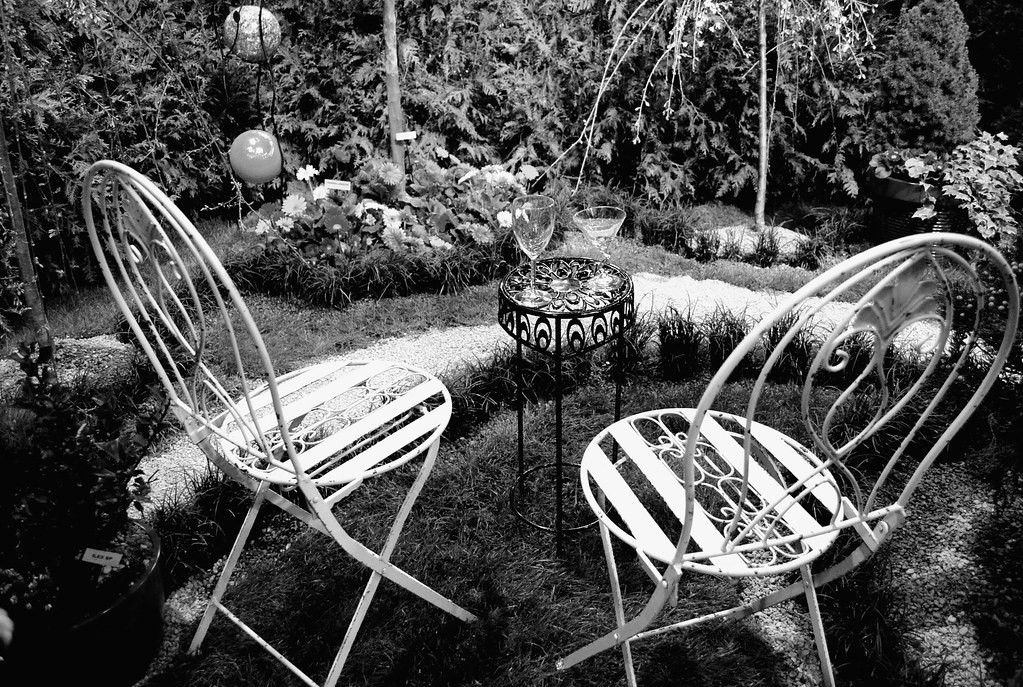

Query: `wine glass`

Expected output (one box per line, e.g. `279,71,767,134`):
511,195,555,308
573,206,626,292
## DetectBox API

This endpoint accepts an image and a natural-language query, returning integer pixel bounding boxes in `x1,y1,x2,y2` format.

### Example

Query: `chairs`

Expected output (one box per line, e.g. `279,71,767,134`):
556,230,1020,687
81,160,478,687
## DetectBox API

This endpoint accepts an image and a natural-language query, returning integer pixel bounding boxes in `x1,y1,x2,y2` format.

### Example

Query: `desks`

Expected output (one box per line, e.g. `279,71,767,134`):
497,258,635,558
